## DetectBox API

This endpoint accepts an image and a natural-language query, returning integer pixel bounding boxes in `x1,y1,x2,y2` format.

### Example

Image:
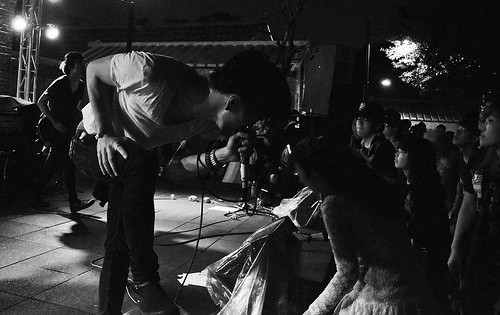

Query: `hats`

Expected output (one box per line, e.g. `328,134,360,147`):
352,101,383,122
382,109,401,123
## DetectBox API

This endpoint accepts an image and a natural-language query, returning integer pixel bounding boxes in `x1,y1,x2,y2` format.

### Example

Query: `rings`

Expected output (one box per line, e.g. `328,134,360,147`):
115,144,120,151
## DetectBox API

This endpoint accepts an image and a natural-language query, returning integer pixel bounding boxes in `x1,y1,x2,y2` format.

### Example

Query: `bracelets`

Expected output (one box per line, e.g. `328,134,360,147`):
205,149,220,169
94,132,107,140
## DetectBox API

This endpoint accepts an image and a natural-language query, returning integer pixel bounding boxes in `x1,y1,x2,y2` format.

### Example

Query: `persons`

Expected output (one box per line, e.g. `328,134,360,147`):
68,49,293,315
37,52,95,213
290,90,500,315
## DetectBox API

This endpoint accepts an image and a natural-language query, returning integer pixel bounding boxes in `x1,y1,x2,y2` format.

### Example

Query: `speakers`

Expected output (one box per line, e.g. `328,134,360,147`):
244,215,302,264
301,42,354,118
280,186,320,228
205,234,290,315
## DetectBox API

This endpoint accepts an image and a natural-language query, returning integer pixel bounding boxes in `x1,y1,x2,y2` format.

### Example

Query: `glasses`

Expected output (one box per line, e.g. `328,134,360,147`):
72,62,83,65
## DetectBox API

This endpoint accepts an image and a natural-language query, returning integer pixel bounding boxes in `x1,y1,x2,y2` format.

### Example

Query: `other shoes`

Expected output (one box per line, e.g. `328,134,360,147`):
70,199,95,213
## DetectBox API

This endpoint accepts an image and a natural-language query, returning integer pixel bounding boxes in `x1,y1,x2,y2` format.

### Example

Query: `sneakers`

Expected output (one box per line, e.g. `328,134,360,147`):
127,280,180,315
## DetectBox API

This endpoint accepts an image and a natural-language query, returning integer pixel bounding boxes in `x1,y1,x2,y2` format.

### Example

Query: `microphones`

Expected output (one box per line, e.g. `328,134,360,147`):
238,136,251,200
